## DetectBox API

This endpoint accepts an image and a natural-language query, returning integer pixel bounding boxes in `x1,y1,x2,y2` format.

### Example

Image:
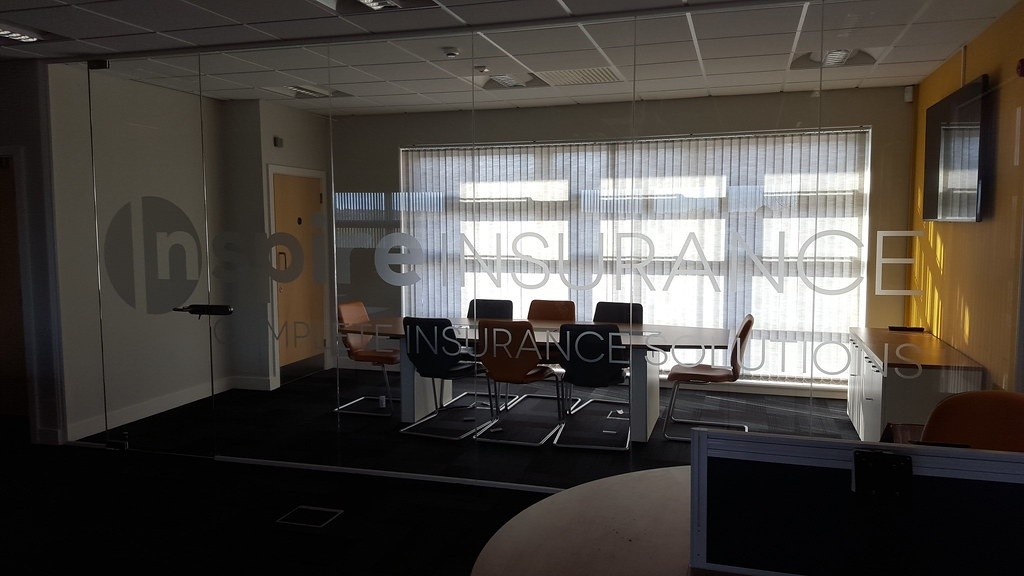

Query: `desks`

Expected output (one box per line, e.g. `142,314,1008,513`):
337,316,729,443
472,466,732,576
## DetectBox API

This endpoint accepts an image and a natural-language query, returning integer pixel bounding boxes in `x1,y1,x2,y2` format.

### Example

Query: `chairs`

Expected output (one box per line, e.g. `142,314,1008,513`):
471,320,562,447
504,300,580,416
333,300,401,417
440,299,520,412
921,390,1024,455
662,314,754,443
553,323,631,450
568,302,643,418
399,316,495,441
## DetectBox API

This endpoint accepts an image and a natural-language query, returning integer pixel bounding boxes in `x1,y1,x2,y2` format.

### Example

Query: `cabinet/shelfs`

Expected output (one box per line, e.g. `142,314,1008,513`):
845,326,988,443
